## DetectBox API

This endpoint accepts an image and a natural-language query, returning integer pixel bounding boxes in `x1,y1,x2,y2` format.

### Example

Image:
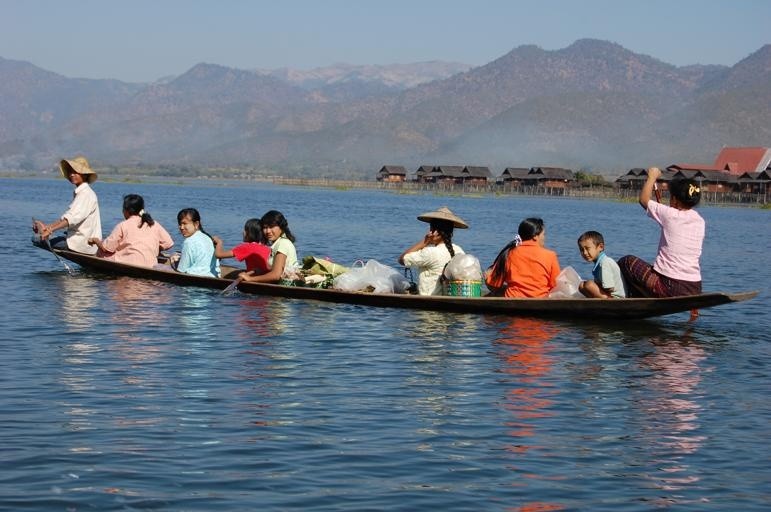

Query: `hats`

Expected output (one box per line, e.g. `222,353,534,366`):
417,207,468,229
59,158,97,185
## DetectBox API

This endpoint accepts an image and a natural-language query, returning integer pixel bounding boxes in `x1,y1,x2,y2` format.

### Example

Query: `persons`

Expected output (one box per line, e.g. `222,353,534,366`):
32,157,103,255
170,208,219,275
398,206,465,296
484,219,560,297
238,211,298,285
577,231,626,299
618,168,705,297
213,219,272,276
88,194,174,267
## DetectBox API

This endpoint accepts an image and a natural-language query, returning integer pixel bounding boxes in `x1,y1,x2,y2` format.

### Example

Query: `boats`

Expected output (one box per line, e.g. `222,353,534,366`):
29,232,761,319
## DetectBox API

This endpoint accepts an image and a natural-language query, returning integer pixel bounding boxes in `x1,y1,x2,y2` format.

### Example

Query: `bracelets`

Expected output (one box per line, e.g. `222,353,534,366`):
45,229,54,235
